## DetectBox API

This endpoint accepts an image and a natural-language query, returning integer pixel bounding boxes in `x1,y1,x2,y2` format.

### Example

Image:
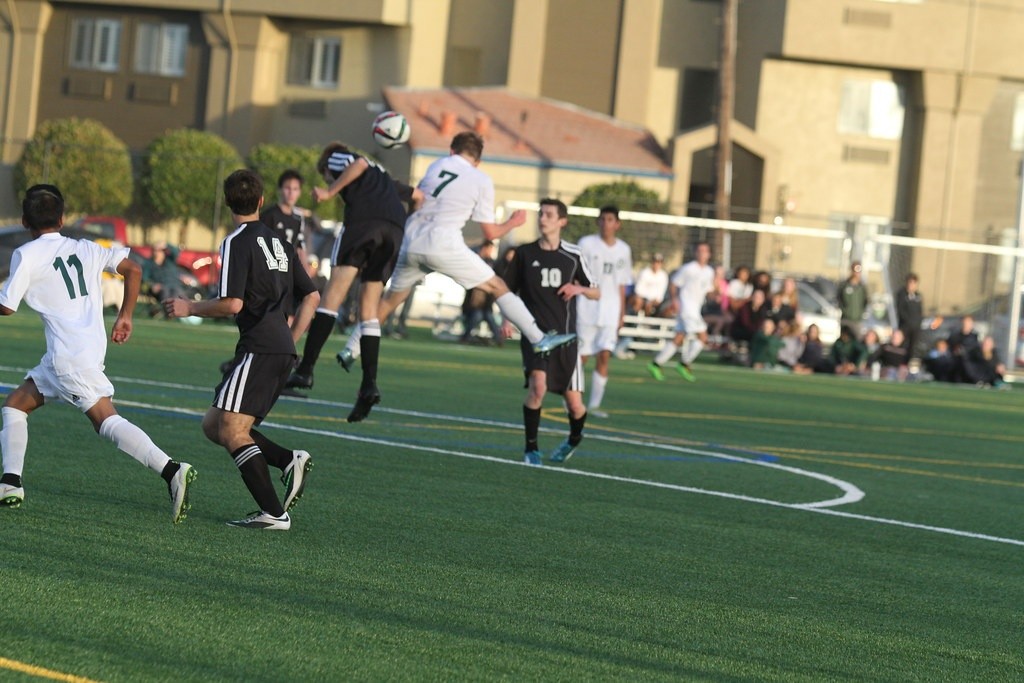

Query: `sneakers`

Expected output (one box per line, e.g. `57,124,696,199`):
530,329,578,358
336,347,354,373
168,460,197,525
0,482,24,509
279,370,314,389
346,386,381,423
523,449,541,468
645,360,664,382
550,437,578,464
225,510,292,532
280,449,315,511
674,363,696,383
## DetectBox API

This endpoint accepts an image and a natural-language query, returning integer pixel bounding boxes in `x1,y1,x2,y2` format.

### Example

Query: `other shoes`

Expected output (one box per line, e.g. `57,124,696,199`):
589,410,609,419
219,358,235,374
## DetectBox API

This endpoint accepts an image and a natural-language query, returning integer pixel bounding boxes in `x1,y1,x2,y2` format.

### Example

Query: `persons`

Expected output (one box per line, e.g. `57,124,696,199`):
612,252,686,360
0,184,198,526
837,261,868,340
833,323,912,382
894,273,925,342
500,196,599,466
337,127,576,371
916,315,1011,389
573,204,635,418
460,241,513,345
699,260,826,375
259,171,314,398
286,135,424,423
647,239,719,382
165,167,315,531
141,240,186,301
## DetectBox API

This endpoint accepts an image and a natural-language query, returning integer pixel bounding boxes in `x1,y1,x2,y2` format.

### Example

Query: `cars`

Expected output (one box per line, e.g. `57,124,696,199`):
0,225,146,287
768,273,893,348
918,291,1024,373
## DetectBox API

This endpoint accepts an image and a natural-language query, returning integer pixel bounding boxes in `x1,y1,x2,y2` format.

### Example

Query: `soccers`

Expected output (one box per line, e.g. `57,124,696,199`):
371,110,412,150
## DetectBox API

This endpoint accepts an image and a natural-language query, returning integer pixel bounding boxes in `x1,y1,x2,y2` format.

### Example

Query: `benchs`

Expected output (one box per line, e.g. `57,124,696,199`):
612,314,738,355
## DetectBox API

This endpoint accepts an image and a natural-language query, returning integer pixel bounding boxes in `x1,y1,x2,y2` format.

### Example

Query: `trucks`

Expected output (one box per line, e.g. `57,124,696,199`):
66,215,222,304
